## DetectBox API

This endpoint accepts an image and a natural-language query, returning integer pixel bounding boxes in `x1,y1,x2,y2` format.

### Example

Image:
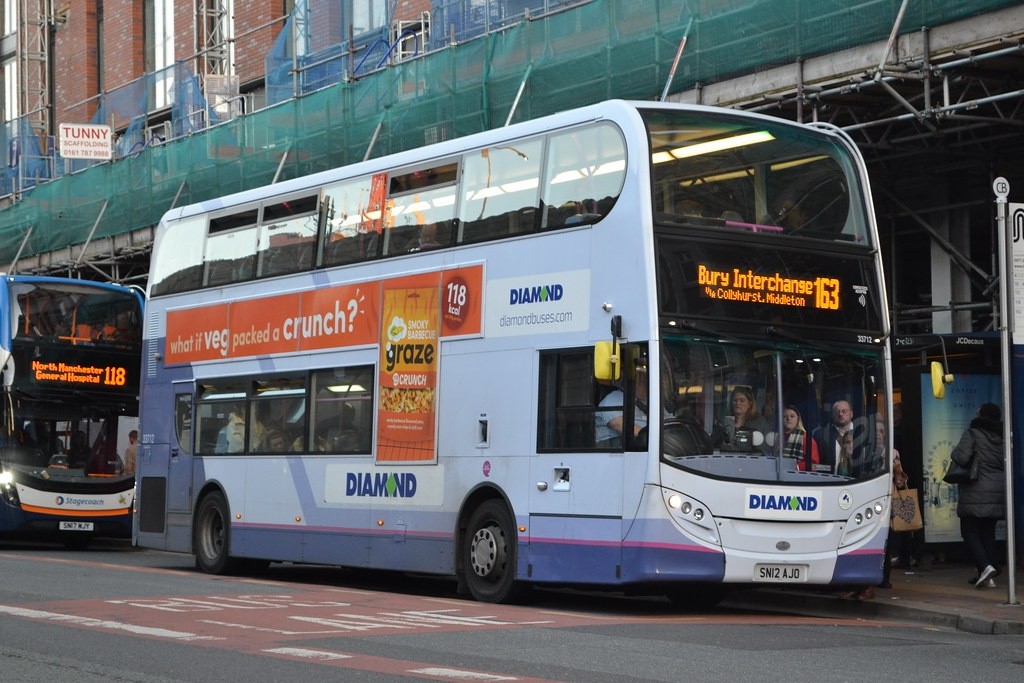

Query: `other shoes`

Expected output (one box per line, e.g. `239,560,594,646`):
973,564,997,589
881,582,892,588
890,554,917,566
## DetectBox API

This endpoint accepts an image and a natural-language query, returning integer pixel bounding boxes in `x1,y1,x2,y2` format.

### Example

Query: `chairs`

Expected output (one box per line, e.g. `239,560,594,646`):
168,229,410,297
259,427,357,451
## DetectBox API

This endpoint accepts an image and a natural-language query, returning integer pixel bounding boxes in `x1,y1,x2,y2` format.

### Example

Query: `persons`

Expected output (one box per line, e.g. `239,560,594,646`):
592,365,677,447
125,429,138,472
951,402,1006,589
712,386,943,600
403,224,443,253
226,401,361,454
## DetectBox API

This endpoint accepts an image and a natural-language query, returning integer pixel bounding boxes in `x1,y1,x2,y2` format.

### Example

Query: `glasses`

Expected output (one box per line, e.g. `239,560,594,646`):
734,385,752,392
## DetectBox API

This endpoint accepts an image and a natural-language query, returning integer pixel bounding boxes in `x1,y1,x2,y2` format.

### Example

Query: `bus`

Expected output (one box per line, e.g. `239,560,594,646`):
0,271,145,547
133,99,954,603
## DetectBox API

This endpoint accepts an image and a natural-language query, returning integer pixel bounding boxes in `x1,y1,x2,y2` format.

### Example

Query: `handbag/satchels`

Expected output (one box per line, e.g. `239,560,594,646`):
890,480,923,531
943,428,979,484
